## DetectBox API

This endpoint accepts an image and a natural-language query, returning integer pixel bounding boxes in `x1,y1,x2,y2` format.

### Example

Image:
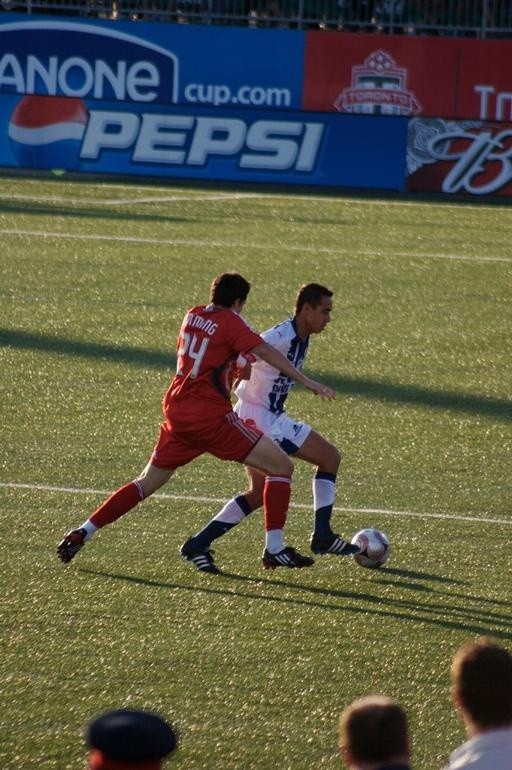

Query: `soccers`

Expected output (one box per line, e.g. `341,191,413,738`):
351,528,391,567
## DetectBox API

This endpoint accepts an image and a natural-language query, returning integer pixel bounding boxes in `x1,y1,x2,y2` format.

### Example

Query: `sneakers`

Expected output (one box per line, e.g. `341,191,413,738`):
262,546,315,570
311,532,360,556
56,527,88,564
179,538,220,574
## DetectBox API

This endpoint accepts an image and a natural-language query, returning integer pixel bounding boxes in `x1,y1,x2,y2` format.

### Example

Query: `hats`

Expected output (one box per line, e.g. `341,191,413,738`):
85,710,176,762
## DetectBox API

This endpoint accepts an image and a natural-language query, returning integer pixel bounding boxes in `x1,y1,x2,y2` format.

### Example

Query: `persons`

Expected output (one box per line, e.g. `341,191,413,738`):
57,274,315,573
179,282,361,573
84,707,178,768
337,638,510,770
